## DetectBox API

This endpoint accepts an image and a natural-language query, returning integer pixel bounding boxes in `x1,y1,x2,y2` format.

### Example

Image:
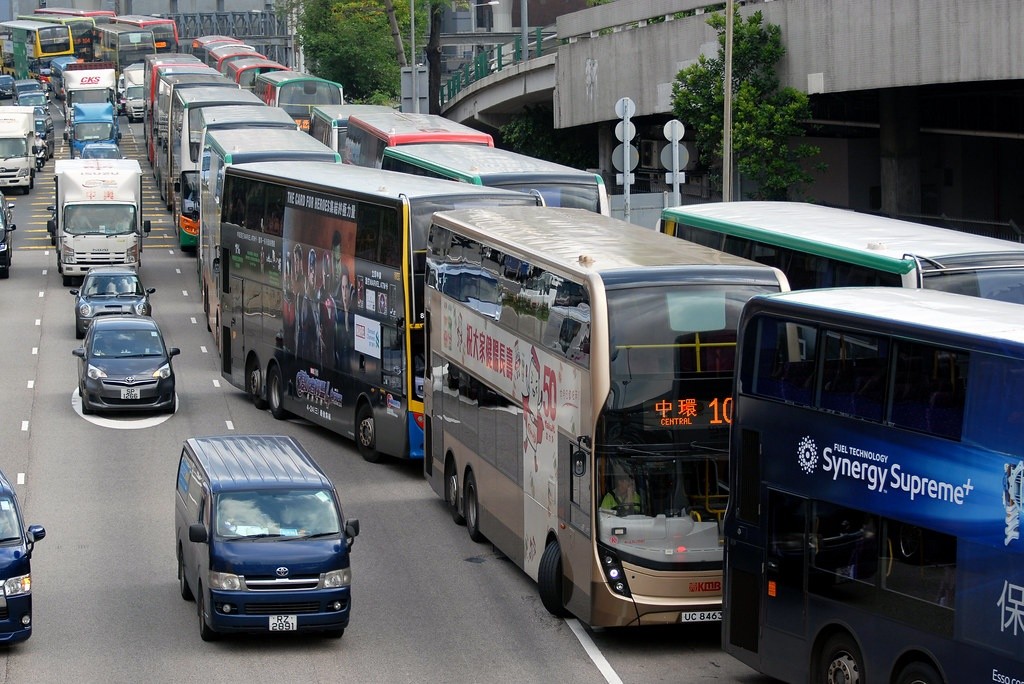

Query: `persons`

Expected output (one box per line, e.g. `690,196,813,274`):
601,476,640,511
283,231,354,371
67,208,92,229
111,212,129,229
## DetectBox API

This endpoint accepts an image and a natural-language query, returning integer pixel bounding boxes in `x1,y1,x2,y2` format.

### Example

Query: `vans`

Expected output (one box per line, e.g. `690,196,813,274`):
50,56,78,101
542,300,591,371
174,435,359,645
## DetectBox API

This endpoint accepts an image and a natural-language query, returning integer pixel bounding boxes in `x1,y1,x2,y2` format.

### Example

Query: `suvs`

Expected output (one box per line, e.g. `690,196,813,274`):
13,78,55,162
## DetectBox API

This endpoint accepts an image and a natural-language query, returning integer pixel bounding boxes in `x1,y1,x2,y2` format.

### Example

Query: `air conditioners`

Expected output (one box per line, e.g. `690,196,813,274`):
641,140,670,170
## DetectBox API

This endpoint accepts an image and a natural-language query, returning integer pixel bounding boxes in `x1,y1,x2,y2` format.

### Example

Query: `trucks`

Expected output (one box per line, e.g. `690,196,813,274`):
47,159,151,287
0,105,39,196
61,61,122,135
117,62,144,124
64,103,122,160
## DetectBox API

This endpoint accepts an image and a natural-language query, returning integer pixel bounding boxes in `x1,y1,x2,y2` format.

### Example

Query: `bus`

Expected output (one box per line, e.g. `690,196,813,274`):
344,112,494,170
421,204,807,633
380,141,611,218
658,197,1024,307
215,160,546,467
195,128,342,359
0,8,183,93
720,286,1024,683
309,105,398,165
143,34,296,254
254,71,343,137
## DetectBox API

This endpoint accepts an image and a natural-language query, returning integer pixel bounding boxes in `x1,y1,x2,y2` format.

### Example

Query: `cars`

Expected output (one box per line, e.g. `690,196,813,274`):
0,75,15,99
430,258,503,324
74,143,128,161
0,190,17,280
0,470,47,648
71,315,180,416
118,75,125,100
69,266,156,340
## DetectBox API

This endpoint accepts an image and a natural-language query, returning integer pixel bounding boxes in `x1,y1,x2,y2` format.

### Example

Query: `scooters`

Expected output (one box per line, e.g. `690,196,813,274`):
34,140,48,173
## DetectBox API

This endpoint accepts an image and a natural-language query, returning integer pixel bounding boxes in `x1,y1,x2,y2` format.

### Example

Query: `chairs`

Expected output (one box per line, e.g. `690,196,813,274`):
777,249,876,290
773,354,966,442
